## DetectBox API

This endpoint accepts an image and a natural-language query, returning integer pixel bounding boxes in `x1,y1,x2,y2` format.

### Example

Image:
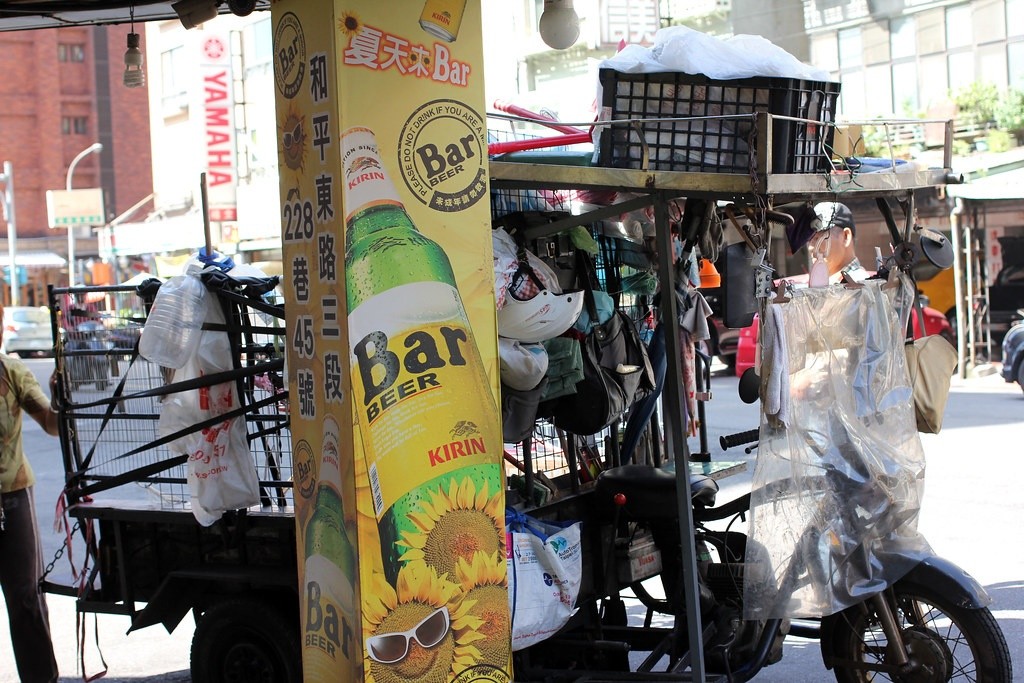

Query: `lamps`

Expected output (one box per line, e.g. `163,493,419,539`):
539,0,581,50
122,0,146,88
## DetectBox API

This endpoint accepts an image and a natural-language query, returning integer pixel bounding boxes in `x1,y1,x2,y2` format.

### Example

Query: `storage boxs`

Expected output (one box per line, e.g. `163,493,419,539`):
596,67,842,176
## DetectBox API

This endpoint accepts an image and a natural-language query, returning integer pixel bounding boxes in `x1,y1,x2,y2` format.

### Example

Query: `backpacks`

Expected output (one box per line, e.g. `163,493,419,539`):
672,531,791,667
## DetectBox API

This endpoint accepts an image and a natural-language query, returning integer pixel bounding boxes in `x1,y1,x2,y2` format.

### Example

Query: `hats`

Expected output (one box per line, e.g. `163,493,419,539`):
814,201,856,235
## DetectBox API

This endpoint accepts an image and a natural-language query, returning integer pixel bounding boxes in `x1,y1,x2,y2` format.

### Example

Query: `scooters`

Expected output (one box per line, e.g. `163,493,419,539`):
61,312,117,391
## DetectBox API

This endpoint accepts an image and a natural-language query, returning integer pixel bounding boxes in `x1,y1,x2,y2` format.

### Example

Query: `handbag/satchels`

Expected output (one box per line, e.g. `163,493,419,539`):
506,516,583,652
903,334,959,434
546,309,656,436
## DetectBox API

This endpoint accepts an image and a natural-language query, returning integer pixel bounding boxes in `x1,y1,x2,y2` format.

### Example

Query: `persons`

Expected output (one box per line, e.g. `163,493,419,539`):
787,202,904,630
0,303,60,683
60,285,114,387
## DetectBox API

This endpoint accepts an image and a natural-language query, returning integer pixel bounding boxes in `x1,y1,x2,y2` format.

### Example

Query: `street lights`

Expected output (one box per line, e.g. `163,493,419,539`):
66,142,103,305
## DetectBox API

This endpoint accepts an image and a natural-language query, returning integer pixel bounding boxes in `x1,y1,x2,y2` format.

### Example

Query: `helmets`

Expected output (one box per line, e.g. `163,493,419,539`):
72,283,88,295
496,288,585,343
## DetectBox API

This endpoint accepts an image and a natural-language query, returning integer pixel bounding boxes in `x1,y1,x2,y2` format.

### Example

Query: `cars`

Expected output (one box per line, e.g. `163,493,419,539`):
735,267,957,382
1,306,54,358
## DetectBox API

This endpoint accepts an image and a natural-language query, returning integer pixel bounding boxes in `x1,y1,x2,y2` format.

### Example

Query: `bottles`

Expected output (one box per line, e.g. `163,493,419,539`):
139,260,209,368
340,127,503,595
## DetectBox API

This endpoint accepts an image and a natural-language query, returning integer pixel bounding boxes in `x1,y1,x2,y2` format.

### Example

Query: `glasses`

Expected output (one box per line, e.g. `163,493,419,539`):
365,606,449,662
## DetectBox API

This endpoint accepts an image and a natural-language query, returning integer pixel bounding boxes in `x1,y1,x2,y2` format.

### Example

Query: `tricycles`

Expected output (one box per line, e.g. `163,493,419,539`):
0,187,1018,682
